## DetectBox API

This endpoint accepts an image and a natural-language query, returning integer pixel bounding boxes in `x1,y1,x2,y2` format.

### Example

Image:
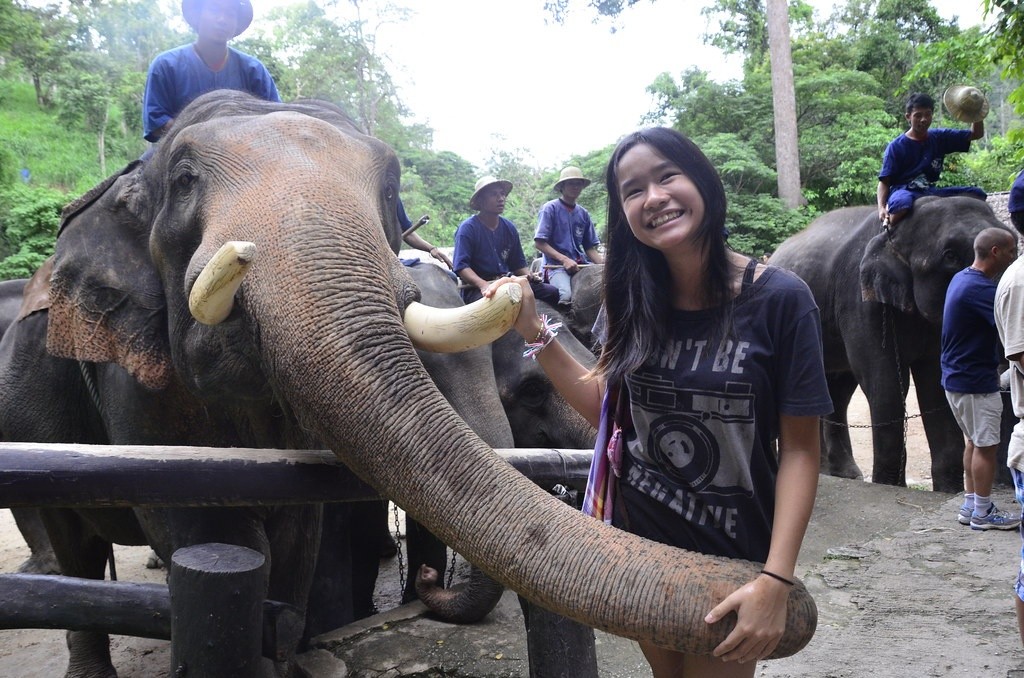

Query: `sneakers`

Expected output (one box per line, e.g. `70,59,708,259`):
971,503,1021,530
957,505,974,524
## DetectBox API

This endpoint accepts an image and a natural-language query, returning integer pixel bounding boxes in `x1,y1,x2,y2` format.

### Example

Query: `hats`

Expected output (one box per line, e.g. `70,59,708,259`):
470,175,512,210
553,166,591,190
181,0,253,37
944,85,989,123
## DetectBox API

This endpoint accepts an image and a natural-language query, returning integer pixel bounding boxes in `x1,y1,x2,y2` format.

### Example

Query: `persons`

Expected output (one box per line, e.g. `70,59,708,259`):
397,196,458,285
534,166,605,312
453,175,560,308
142,0,281,161
877,94,988,232
483,128,835,678
940,228,1024,529
993,170,1024,646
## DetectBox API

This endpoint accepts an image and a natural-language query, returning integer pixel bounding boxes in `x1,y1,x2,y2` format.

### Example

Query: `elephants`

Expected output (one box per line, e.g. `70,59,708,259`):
0,89,819,678
764,194,1018,493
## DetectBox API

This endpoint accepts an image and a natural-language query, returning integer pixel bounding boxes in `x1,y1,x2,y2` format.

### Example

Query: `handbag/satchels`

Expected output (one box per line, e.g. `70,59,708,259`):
581,384,623,523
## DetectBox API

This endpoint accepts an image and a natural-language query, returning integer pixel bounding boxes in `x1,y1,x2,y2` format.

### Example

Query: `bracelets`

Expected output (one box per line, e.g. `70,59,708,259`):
760,569,795,586
428,246,438,252
523,315,563,361
